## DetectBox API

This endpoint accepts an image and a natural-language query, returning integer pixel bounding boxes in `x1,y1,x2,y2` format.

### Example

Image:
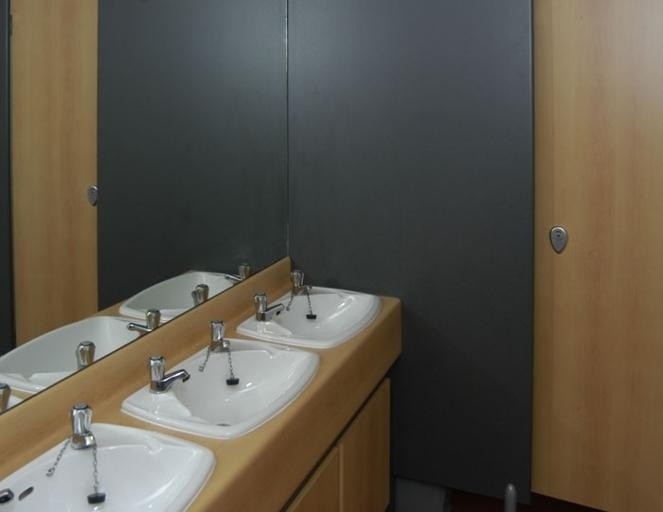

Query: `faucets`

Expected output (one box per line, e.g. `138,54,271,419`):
0,485,34,512
0,383,10,414
224,263,253,285
126,308,162,335
75,340,96,371
190,284,209,306
209,320,230,353
253,292,285,321
289,269,311,297
146,353,190,392
70,403,97,450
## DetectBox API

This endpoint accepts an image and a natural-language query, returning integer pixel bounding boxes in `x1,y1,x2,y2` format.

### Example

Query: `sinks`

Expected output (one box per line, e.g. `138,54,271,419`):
0,424,218,511
0,314,163,394
118,270,238,319
235,286,384,348
119,337,321,439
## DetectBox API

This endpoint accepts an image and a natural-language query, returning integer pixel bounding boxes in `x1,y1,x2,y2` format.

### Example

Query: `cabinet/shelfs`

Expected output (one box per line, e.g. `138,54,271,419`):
285,378,391,512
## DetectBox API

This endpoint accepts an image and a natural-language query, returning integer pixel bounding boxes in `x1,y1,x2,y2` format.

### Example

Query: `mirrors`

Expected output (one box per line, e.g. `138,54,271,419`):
1,0,288,416
